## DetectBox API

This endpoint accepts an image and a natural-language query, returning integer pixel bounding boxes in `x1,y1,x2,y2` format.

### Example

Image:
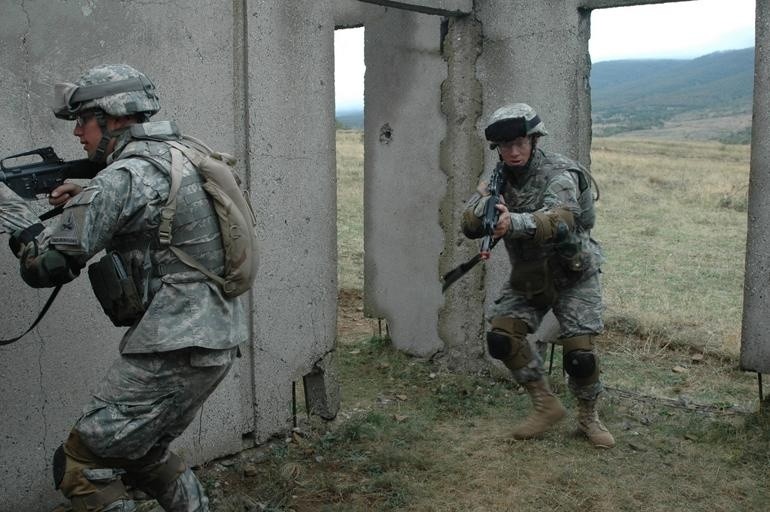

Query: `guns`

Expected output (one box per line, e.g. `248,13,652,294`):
0,147,91,198
480,162,507,260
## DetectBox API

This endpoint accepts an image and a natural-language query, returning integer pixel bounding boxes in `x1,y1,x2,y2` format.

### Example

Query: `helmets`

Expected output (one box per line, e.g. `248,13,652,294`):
485,103,549,150
53,63,161,120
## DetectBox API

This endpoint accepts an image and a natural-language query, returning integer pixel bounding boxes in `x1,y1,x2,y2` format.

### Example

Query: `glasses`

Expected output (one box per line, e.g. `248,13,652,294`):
75,106,104,128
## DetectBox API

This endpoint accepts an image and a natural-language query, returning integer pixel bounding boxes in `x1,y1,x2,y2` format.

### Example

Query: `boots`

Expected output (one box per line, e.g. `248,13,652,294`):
578,393,616,448
513,375,567,440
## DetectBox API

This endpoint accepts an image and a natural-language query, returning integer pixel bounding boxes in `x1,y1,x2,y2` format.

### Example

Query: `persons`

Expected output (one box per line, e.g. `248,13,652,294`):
462,102,616,448
0,65,252,512
